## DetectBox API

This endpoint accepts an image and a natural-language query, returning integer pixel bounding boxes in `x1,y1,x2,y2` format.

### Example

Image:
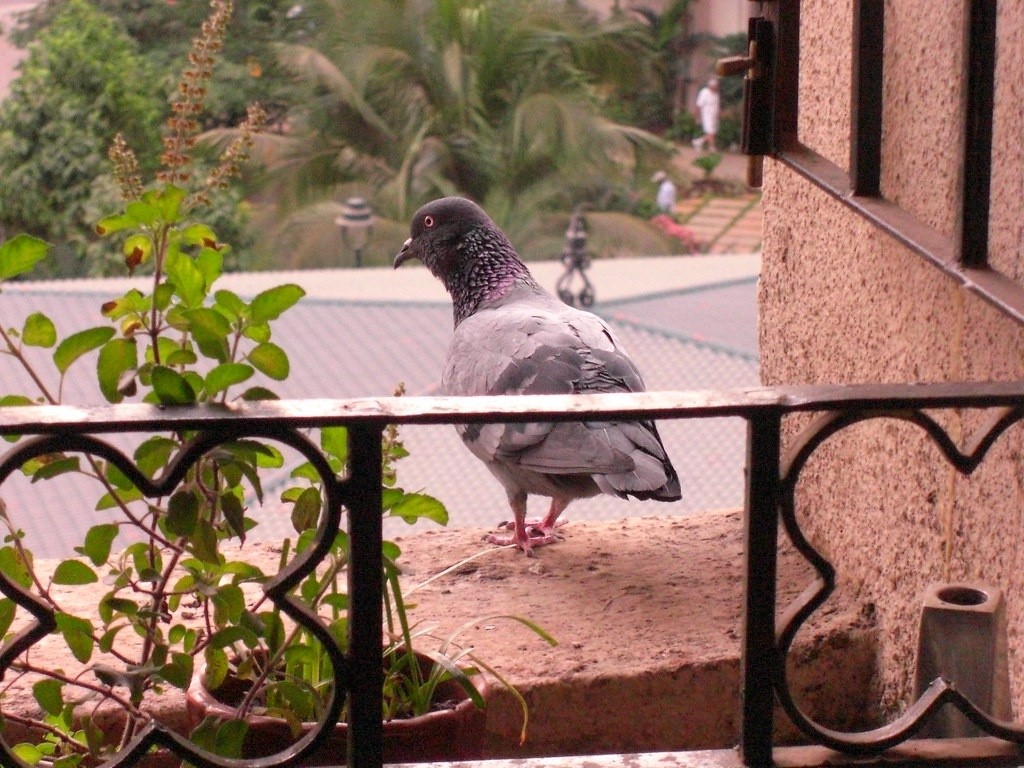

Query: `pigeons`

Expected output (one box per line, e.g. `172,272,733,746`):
391,196,683,559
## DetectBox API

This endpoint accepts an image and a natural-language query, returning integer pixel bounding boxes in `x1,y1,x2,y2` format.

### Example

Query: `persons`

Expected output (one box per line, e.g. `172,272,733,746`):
651,170,675,215
691,78,720,153
